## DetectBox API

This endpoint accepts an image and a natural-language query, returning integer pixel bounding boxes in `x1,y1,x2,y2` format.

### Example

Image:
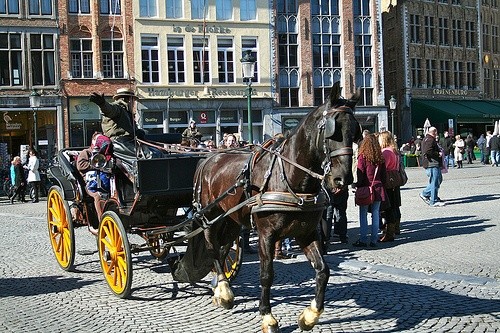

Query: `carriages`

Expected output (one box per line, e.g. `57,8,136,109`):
46,80,363,333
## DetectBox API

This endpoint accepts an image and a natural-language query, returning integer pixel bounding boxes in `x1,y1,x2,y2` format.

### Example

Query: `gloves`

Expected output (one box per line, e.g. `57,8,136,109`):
89,92,106,106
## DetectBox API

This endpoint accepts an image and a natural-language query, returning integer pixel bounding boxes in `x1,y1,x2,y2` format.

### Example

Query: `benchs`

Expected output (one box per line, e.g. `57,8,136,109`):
57,148,136,203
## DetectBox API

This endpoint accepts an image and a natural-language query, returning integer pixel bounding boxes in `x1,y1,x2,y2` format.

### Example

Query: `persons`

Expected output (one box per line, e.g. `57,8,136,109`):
76,131,120,220
177,133,248,152
182,120,199,139
8,145,40,204
349,125,476,246
477,130,500,167
89,88,170,196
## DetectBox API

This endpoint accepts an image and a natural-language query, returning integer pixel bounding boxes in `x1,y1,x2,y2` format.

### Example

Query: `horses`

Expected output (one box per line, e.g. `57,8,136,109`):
191,81,362,333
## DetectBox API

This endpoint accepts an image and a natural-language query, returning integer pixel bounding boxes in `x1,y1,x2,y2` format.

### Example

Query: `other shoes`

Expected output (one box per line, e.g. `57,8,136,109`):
325,221,400,247
33,197,38,202
430,201,445,206
418,192,430,205
434,197,444,202
238,226,297,257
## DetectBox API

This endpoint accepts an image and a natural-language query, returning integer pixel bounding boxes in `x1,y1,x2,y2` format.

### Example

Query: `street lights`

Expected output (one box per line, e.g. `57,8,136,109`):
239,50,257,146
388,95,397,138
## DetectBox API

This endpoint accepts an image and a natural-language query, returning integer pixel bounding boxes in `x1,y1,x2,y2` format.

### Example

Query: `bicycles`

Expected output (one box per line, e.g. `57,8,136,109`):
0,168,46,199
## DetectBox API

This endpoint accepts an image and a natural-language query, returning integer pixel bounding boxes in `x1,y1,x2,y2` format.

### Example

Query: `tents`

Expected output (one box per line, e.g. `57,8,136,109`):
411,99,500,137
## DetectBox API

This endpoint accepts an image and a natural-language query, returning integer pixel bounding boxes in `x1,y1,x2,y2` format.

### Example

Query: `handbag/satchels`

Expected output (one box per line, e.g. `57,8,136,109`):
384,169,407,191
440,159,449,173
460,148,465,155
355,186,374,205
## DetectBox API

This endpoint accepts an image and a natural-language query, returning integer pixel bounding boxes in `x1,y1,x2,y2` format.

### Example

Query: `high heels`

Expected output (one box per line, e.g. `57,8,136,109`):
10,197,13,204
21,198,28,203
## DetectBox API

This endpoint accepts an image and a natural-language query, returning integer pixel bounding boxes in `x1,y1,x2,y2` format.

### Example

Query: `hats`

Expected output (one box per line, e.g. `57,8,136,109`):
112,87,139,101
